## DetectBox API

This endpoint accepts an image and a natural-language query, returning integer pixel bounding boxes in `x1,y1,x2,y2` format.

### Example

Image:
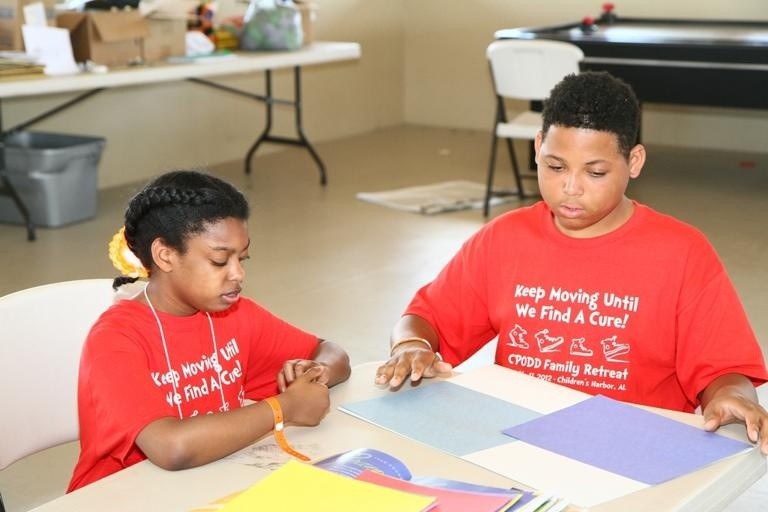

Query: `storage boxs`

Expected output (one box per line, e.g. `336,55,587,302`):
0,0,71,51
140,10,197,61
56,10,146,66
0,128,105,229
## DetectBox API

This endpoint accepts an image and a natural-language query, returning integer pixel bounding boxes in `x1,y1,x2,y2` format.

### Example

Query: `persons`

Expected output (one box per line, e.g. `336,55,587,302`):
373,69,768,460
66,170,354,500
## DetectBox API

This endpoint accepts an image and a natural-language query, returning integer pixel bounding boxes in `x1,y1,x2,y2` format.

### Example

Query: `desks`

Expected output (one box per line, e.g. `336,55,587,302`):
493,18,767,170
32,354,767,512
0,41,361,243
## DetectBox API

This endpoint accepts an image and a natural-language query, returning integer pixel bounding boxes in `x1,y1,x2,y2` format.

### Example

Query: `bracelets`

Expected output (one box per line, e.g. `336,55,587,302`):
263,394,287,435
388,336,434,356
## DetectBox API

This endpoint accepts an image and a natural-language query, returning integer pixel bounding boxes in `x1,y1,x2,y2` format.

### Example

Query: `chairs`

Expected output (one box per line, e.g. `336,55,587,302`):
1,277,151,512
483,39,585,217
